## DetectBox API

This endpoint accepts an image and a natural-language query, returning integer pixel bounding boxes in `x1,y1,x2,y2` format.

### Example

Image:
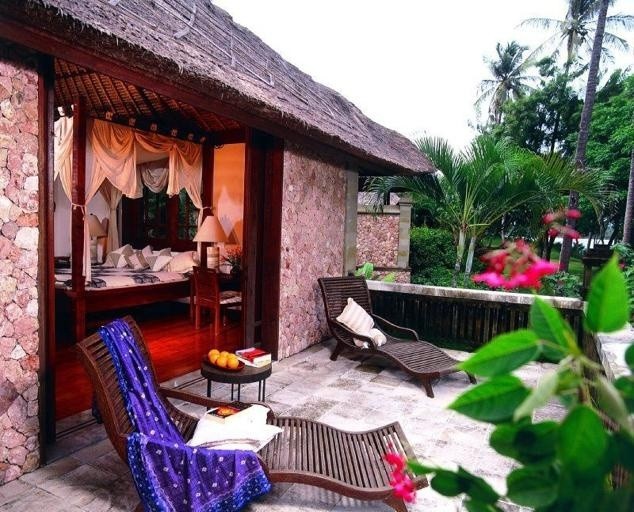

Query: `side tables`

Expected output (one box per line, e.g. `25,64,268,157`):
201,361,271,403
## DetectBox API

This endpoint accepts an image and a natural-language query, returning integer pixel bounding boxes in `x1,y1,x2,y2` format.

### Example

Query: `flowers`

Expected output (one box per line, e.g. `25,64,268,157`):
220,247,242,266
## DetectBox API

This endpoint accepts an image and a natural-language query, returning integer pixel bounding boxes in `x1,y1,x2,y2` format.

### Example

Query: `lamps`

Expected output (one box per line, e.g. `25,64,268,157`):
88,212,105,263
192,216,228,273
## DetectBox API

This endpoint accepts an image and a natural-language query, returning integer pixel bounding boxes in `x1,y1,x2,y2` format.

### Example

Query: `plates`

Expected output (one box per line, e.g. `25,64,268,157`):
208,355,245,372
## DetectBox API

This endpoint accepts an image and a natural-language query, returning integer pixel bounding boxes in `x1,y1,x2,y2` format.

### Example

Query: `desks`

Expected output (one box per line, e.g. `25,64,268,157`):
188,273,241,327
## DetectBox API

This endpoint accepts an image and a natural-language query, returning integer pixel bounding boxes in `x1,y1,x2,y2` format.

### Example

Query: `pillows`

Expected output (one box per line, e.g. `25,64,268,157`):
352,328,386,349
186,404,283,454
102,244,197,274
337,298,375,335
132,273,160,283
65,278,106,287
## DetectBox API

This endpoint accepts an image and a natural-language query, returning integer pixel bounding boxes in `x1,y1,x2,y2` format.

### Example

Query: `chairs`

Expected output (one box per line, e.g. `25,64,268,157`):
75,314,428,512
193,266,242,334
319,275,476,399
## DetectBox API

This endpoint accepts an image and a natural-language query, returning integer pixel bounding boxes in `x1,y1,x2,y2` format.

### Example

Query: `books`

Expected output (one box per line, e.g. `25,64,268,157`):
235,346,271,365
204,400,253,424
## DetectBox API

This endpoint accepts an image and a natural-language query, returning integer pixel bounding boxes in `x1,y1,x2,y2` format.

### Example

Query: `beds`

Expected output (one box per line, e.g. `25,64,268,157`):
54,97,213,353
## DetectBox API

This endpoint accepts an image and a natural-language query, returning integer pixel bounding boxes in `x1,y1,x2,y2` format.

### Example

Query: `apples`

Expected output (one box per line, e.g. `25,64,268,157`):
227,358,239,369
209,349,220,357
220,351,229,359
208,353,220,365
216,356,226,368
227,353,236,360
